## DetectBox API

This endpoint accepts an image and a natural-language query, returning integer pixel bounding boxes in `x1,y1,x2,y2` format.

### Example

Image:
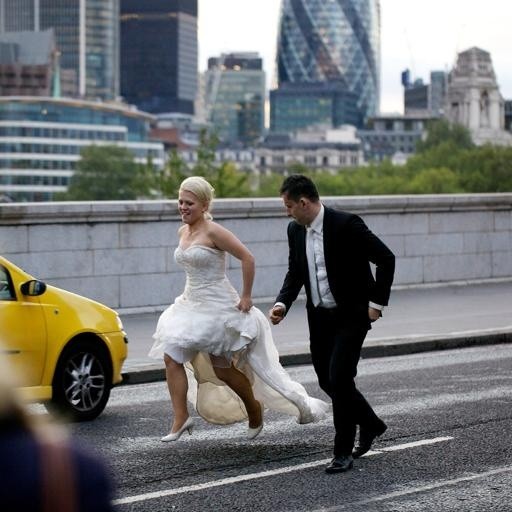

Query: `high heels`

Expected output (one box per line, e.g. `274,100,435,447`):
159,414,193,441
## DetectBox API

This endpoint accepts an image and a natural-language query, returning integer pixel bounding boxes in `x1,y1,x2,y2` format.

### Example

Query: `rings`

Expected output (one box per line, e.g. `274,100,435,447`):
249,304,252,307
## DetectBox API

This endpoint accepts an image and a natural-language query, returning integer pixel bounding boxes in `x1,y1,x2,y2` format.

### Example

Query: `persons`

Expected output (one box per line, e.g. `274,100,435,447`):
147,174,330,444
1,341,117,512
267,175,397,474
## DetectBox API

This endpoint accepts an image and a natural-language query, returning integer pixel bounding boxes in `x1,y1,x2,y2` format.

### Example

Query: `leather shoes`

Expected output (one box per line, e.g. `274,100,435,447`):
324,454,355,473
353,417,387,460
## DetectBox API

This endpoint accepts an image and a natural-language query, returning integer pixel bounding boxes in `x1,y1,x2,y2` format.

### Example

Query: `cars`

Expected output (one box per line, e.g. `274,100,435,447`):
0,255,128,422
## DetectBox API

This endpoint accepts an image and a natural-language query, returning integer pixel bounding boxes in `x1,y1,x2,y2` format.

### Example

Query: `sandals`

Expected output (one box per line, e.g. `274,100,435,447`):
245,398,264,439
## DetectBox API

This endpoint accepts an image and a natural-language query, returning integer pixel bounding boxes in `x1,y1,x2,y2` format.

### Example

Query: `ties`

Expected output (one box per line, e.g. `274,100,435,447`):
304,226,321,307
